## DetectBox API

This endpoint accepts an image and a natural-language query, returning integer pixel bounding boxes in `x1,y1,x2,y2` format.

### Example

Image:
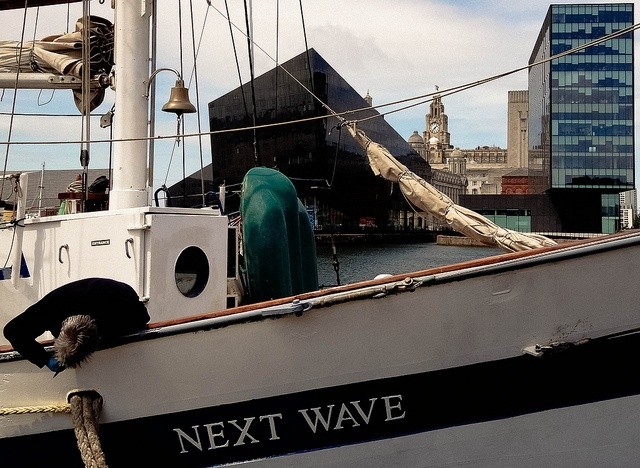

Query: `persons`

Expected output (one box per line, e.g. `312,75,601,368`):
3,278,150,373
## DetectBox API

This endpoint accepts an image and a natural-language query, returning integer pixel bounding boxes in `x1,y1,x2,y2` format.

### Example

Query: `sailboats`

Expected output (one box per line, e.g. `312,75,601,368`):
1,1,640,468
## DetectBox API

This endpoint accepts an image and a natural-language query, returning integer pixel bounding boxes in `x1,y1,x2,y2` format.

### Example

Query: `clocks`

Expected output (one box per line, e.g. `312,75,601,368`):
429,121,440,134
